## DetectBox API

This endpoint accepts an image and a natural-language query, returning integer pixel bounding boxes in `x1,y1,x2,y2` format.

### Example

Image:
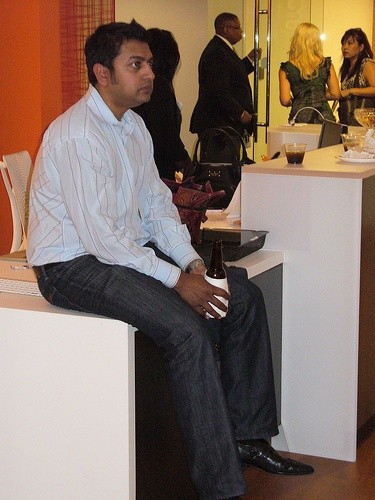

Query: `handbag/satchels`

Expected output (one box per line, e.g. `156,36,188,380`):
278,106,325,152
186,126,255,209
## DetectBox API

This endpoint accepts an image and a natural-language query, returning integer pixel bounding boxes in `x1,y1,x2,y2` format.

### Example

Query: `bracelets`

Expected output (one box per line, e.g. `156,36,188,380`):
185,259,205,274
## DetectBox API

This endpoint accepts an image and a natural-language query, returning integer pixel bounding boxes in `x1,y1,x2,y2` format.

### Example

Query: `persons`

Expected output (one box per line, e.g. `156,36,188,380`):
338,27,375,144
130,28,191,193
27,23,314,500
278,23,341,124
189,13,263,164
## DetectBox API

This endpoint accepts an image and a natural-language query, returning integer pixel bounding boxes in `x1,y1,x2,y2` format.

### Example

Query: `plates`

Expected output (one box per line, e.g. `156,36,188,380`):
335,154,375,163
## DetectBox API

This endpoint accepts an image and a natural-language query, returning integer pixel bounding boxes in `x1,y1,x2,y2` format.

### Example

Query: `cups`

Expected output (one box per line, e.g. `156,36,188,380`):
354,108,375,127
284,142,307,165
339,126,375,154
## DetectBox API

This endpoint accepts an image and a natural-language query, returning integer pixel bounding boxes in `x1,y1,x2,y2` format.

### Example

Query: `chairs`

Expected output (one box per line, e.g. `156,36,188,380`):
0,151,34,253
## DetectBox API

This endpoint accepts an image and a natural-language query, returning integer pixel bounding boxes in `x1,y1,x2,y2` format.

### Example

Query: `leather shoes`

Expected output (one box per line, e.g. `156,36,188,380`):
235,442,315,477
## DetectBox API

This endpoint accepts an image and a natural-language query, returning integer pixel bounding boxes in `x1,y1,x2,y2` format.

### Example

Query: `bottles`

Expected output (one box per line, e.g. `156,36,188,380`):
204,239,230,319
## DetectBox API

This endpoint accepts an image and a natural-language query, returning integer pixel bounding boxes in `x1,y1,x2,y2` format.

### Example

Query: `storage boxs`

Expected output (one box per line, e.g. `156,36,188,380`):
193,228,269,262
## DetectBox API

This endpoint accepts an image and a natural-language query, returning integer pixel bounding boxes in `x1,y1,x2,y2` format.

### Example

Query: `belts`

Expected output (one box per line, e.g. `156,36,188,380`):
31,262,59,277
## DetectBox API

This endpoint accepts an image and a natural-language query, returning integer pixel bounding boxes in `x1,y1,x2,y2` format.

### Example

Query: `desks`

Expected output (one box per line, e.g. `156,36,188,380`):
0,248,285,500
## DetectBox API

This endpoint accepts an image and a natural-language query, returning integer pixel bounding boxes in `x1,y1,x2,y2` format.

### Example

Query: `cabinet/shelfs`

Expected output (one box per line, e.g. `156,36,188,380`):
241,144,375,462
